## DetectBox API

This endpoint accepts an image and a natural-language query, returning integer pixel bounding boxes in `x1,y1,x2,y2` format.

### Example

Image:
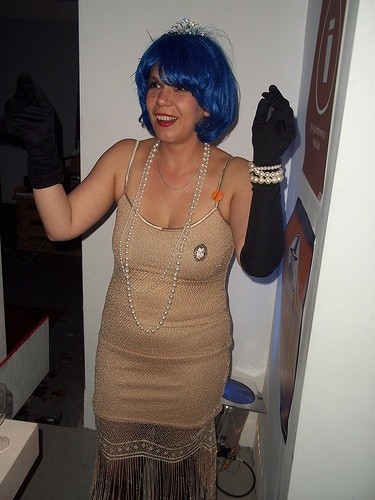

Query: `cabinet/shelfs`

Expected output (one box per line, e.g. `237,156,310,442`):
11,184,81,257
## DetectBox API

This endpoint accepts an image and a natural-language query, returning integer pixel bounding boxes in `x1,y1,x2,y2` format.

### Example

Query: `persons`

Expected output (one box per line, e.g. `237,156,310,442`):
3,18,297,500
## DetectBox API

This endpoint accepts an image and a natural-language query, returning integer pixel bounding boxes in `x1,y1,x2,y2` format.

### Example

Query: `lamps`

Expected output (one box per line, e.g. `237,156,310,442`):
215,377,267,472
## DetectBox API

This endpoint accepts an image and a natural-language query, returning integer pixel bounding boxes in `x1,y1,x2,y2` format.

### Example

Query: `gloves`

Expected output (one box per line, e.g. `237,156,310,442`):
240,85,296,278
2,73,65,189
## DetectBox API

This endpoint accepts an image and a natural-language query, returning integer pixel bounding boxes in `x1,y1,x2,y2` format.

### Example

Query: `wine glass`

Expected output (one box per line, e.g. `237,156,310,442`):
0,383,10,454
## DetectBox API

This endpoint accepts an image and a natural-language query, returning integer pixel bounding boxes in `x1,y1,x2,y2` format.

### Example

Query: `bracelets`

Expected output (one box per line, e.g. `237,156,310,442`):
248,161,285,184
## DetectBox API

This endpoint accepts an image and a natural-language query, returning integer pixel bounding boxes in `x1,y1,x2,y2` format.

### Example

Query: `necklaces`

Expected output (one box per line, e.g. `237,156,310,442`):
119,140,210,334
